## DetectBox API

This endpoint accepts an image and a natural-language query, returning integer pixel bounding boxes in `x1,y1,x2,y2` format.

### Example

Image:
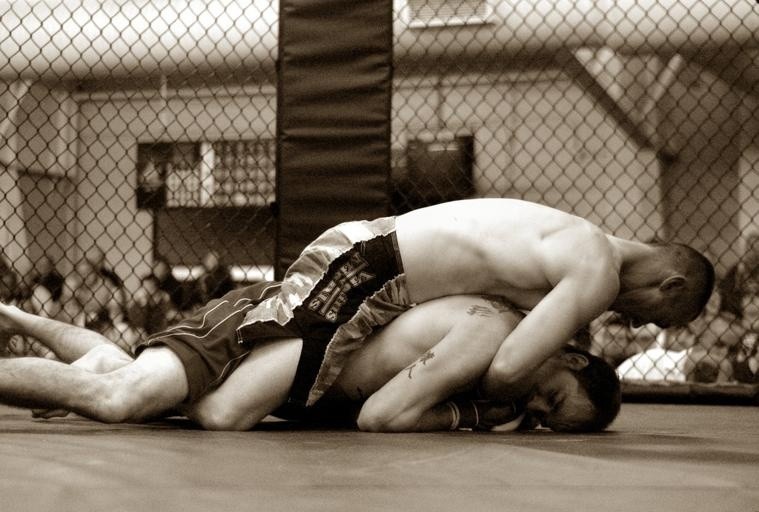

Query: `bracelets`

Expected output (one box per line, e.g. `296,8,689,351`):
472,401,479,425
446,401,460,430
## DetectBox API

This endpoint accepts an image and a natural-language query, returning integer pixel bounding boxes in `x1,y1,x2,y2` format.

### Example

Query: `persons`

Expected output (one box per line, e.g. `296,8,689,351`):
1,249,236,358
33,199,715,432
567,234,758,384
0,280,622,432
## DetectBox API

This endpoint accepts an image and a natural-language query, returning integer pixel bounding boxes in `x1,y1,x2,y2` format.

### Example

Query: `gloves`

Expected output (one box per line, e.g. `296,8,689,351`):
449,395,527,434
